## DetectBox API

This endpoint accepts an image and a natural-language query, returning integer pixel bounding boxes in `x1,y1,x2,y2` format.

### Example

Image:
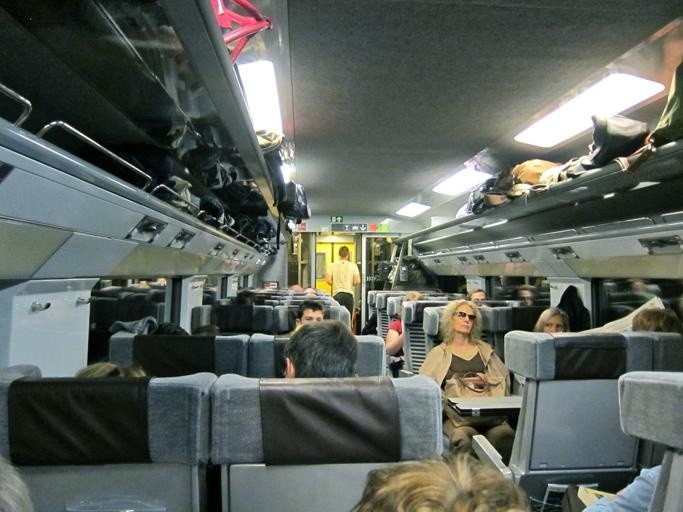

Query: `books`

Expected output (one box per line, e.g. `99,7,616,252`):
577,486,616,507
572,298,664,332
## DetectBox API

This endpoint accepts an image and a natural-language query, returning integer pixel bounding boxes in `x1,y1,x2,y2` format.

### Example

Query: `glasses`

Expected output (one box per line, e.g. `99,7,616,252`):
452,311,476,321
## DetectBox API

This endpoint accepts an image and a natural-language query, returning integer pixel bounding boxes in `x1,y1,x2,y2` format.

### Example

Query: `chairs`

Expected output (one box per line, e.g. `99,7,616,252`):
0,287,682,512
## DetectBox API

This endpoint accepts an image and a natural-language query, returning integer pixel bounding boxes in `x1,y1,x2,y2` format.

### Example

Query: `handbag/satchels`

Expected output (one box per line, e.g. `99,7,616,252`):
464,63,682,212
120,120,315,259
442,370,512,429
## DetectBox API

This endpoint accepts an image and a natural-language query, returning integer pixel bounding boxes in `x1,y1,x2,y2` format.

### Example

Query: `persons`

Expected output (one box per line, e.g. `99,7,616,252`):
531,307,571,333
417,299,516,465
579,463,665,511
629,308,681,333
324,246,360,318
510,288,532,307
350,454,531,512
